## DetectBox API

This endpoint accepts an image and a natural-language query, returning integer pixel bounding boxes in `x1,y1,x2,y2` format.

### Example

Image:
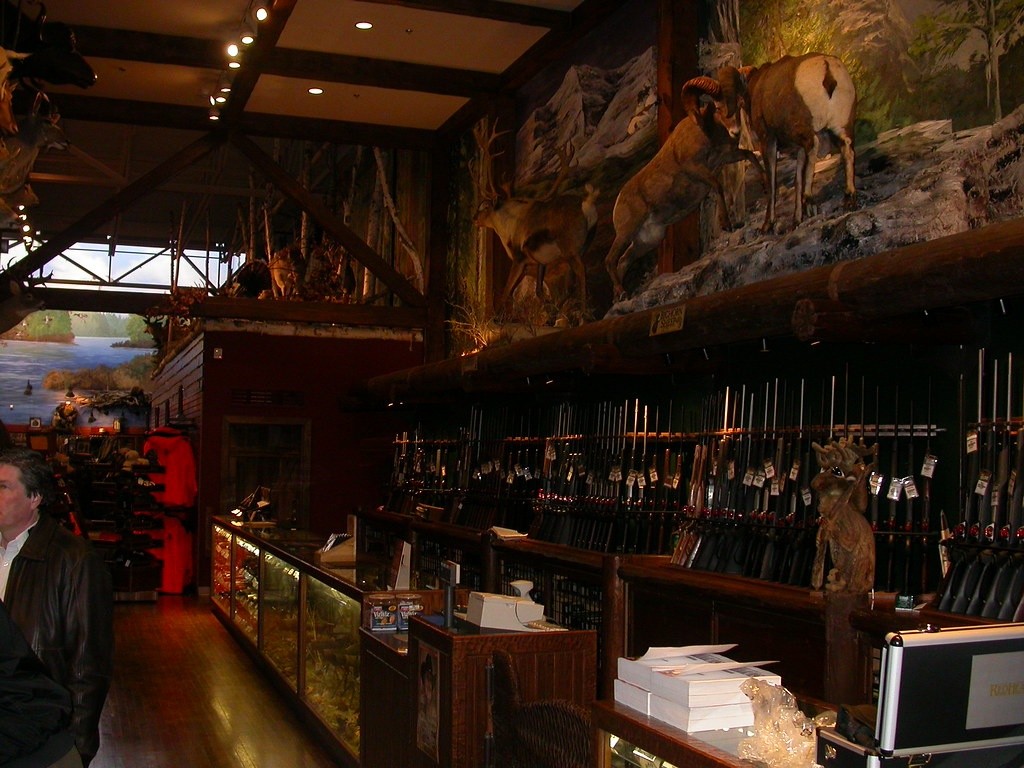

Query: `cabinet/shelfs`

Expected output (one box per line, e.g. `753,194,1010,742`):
354,219,1023,703
407,614,598,767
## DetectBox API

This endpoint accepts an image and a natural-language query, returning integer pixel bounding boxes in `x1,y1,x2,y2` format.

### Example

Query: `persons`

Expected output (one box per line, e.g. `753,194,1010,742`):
0,448,115,768
0,599,88,768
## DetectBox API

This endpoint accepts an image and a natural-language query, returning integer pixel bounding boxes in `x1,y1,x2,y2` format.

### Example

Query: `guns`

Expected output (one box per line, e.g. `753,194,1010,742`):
388,346,1024,623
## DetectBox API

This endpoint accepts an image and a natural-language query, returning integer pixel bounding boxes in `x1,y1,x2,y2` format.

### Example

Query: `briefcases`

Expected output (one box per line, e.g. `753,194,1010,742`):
815,622,1024,768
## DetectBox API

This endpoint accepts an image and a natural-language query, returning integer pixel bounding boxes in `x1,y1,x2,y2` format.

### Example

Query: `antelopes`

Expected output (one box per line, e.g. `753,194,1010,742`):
606,52,858,301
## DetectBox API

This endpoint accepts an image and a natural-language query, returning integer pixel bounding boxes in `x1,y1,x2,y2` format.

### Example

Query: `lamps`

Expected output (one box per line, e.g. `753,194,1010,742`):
65,384,74,397
87,405,100,422
23,380,31,396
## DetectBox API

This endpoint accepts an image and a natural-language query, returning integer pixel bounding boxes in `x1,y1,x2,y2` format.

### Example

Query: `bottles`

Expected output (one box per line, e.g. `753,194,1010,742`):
396,594,424,630
365,594,397,630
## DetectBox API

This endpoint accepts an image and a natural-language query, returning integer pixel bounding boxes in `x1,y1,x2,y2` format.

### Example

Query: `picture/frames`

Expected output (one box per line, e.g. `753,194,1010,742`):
29,417,40,428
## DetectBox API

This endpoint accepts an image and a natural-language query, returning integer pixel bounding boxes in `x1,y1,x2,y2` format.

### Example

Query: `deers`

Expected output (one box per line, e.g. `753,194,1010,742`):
468,116,600,319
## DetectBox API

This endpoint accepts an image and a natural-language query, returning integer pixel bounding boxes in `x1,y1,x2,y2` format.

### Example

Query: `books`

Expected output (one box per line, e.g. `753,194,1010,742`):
613,644,782,733
